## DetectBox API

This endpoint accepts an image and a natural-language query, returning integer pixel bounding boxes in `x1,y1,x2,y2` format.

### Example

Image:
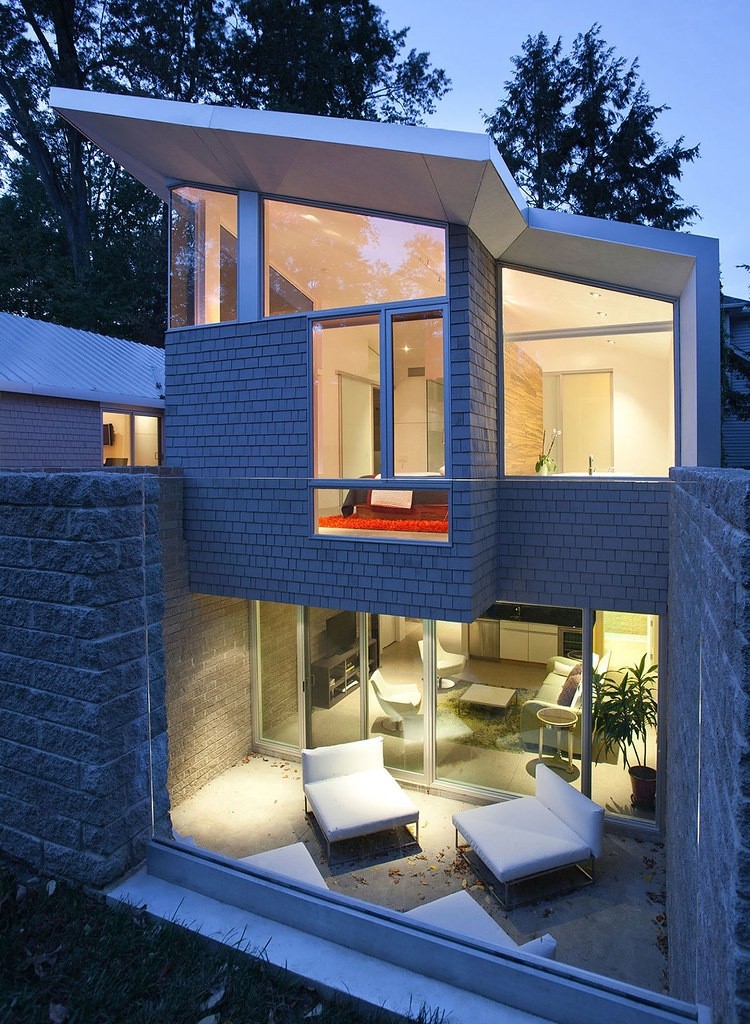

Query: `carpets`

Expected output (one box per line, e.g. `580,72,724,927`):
318,513,447,533
437,679,539,755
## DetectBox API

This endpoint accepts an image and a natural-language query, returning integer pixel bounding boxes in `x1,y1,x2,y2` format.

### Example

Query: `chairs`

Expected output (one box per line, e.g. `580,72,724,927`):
238,842,331,890
371,669,422,732
419,637,466,690
402,890,557,961
302,736,421,866
457,761,607,912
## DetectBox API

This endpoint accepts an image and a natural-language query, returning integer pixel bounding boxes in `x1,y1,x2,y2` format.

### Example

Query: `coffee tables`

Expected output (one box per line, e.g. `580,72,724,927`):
459,684,518,722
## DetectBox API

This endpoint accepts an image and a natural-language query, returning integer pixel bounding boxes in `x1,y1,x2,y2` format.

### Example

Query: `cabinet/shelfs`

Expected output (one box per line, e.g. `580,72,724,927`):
468,616,500,662
310,638,378,709
499,619,559,667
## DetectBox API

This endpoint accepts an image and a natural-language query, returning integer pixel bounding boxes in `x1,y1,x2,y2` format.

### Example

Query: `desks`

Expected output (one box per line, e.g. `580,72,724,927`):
537,707,579,774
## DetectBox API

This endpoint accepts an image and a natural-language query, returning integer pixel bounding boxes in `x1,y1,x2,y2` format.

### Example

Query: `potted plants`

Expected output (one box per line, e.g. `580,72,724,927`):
578,652,658,801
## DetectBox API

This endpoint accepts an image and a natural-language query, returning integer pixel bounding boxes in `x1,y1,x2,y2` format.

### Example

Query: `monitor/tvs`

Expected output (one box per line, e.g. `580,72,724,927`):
103,423,113,446
325,610,356,655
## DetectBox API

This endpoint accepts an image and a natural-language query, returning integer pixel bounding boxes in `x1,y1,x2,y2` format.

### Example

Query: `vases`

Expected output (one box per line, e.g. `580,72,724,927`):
537,464,547,476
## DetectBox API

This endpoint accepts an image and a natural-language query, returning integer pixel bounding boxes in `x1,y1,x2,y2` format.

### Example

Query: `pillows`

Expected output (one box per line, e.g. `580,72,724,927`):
556,674,580,706
439,466,445,476
566,663,582,680
554,661,574,676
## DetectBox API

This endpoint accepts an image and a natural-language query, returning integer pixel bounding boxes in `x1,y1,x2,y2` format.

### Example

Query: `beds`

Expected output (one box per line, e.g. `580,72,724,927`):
343,473,449,522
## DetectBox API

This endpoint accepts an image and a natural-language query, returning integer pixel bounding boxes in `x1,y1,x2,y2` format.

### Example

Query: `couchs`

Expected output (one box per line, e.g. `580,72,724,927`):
520,649,613,759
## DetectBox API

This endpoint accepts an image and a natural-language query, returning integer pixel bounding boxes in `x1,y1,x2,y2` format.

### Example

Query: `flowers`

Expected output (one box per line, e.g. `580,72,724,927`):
535,429,562,472
